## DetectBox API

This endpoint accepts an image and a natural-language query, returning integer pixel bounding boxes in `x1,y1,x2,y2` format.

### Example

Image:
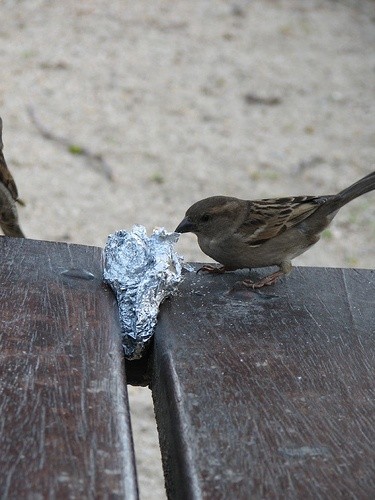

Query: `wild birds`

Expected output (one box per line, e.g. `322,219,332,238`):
174,171,375,292
